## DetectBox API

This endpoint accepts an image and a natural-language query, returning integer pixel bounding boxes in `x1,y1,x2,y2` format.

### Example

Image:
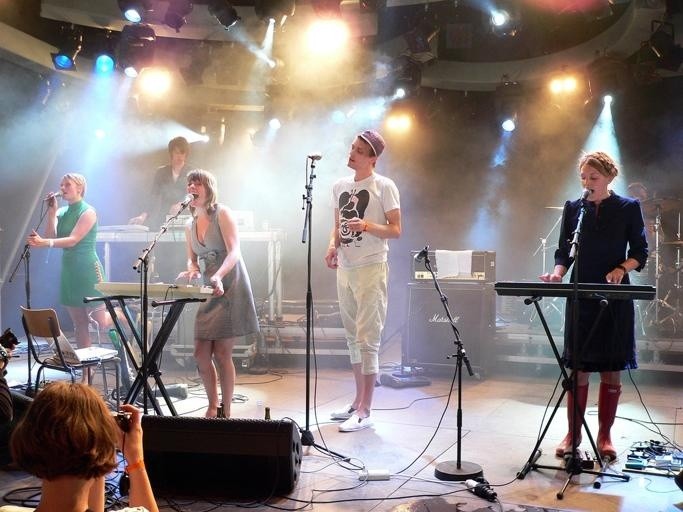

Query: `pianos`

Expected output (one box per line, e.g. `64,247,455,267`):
493,280,657,301
93,281,219,299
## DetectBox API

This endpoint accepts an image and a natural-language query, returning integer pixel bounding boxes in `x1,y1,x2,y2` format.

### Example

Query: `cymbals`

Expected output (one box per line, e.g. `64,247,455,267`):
640,195,680,216
545,205,565,212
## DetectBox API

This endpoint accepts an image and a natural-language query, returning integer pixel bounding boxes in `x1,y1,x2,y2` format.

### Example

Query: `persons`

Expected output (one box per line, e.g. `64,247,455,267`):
324,129,403,431
0,343,16,450
176,169,260,418
11,381,159,512
128,136,194,232
26,173,129,385
538,151,649,459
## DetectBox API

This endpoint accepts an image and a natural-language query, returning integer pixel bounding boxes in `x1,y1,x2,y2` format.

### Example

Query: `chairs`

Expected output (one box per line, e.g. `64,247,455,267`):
16,304,122,415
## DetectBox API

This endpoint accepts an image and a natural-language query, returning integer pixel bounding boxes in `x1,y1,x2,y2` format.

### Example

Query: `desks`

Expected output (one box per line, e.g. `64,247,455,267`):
87,227,287,323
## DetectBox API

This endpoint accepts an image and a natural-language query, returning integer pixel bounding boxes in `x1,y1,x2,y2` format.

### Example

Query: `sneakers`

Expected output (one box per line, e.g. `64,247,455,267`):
338,415,373,433
331,406,356,419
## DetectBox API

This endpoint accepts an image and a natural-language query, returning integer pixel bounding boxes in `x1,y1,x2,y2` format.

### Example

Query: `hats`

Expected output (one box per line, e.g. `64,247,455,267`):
357,128,385,167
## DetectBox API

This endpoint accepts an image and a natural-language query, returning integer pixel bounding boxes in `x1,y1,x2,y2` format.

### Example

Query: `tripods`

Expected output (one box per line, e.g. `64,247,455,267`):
648,248,683,326
644,208,683,336
301,160,352,462
534,204,630,499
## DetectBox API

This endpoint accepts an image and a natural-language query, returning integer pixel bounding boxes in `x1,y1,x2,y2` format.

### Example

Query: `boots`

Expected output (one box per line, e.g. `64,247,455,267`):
597,382,620,457
555,382,590,457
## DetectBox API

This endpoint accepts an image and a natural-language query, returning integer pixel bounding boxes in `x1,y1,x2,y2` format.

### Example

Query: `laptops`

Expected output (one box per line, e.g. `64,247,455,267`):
44,328,118,366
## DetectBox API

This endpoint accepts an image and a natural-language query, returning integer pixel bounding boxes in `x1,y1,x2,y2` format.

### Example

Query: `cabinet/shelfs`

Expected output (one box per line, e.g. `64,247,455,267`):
403,280,496,372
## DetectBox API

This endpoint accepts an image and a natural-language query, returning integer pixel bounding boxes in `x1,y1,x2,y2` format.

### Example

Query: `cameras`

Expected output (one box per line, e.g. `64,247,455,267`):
111,410,130,434
0,328,20,358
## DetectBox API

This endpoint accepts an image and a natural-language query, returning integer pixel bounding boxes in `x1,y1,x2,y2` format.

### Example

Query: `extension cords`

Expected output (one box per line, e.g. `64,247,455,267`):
358,469,391,482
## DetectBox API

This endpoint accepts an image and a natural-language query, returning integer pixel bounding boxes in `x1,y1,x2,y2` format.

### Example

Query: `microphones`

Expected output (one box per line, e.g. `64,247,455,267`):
580,189,590,201
44,190,63,203
181,193,194,205
414,246,428,262
309,153,322,160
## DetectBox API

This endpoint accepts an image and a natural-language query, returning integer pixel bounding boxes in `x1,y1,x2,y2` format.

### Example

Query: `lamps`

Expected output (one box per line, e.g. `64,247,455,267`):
263,56,288,72
179,45,211,86
490,90,520,131
376,54,425,100
399,1,462,53
50,27,83,72
589,57,624,105
359,0,387,15
488,9,522,39
638,18,683,74
114,0,142,25
160,1,193,31
254,0,295,29
89,36,116,74
206,3,241,32
115,24,154,76
